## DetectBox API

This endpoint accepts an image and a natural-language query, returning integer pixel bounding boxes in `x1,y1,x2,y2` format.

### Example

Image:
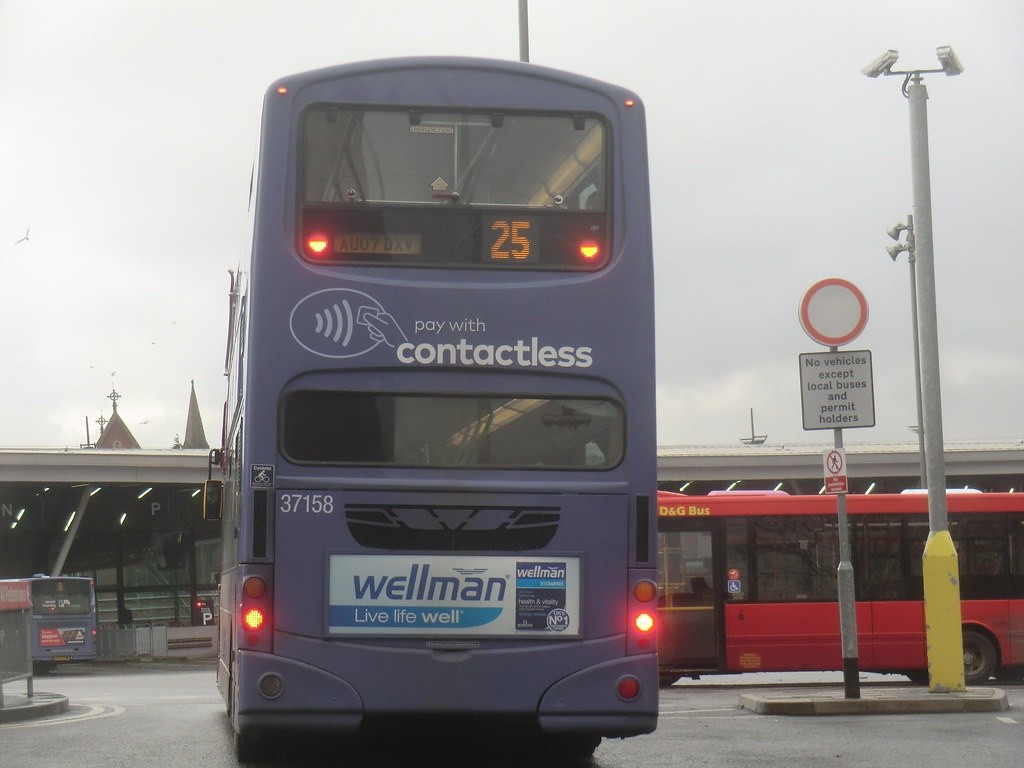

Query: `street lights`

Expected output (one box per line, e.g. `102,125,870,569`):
888,212,925,488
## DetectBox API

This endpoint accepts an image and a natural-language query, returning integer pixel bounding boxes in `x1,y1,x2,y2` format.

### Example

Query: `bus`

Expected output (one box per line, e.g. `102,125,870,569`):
213,55,660,768
655,488,1024,692
23,572,98,679
